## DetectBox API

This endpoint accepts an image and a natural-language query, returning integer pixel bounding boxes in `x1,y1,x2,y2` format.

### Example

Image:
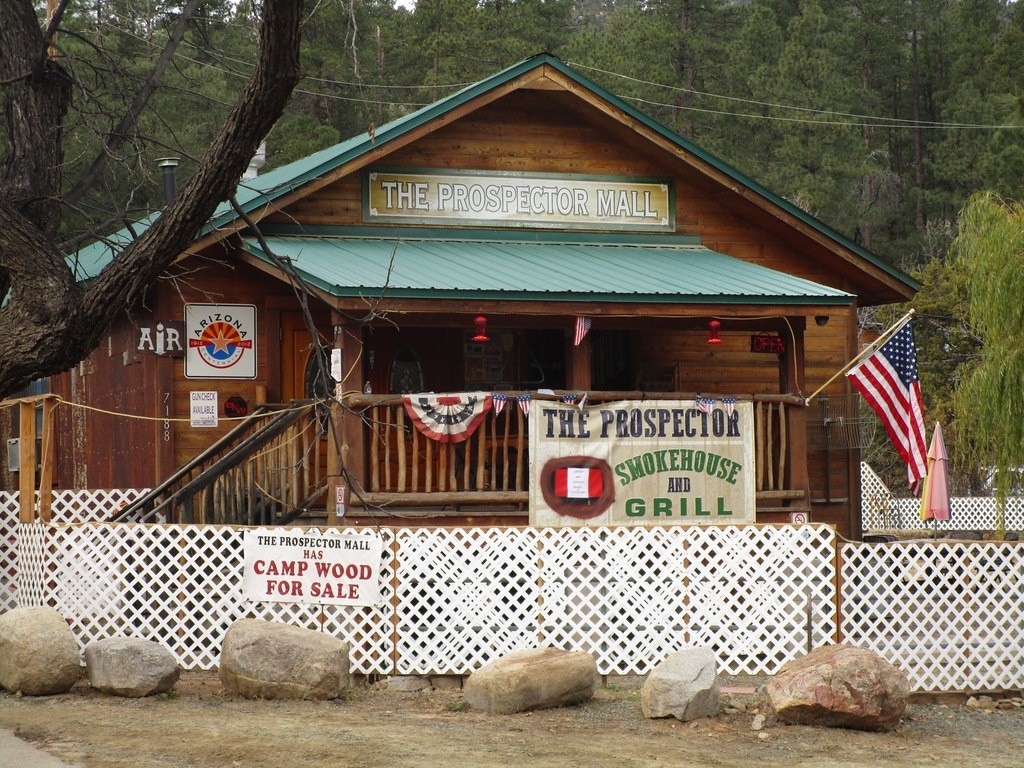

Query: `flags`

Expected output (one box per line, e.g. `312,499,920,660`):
844,316,930,500
574,316,591,346
